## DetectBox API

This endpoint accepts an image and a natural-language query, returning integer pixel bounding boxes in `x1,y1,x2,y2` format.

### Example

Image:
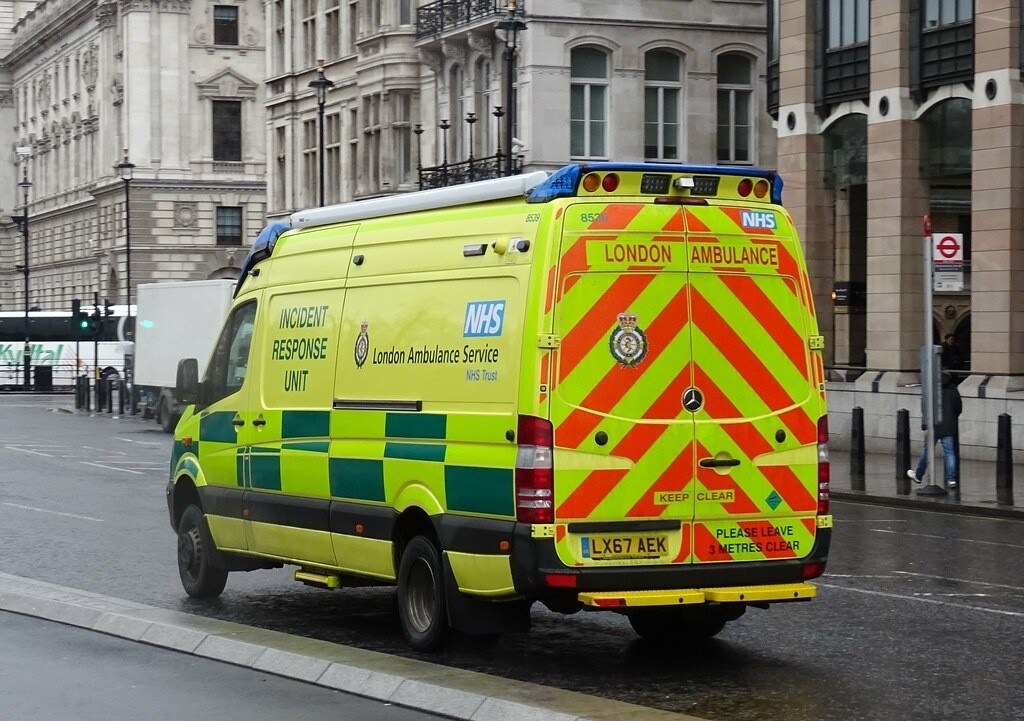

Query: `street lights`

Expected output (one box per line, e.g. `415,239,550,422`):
307,59,336,207
118,147,136,384
495,0,529,177
18,169,34,391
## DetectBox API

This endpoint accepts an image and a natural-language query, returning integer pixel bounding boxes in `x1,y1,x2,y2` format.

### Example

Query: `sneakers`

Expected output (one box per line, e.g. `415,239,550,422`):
906,469,922,484
947,479,957,488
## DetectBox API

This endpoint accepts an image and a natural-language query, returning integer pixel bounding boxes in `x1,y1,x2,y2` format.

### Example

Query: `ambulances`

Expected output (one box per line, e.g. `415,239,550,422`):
165,157,835,653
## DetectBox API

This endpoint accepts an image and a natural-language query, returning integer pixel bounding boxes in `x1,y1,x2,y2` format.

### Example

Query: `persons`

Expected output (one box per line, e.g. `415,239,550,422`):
906,367,963,489
941,332,965,370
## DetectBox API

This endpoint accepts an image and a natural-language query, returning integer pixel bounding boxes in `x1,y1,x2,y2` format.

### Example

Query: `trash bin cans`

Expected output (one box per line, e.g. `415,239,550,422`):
33,364,53,391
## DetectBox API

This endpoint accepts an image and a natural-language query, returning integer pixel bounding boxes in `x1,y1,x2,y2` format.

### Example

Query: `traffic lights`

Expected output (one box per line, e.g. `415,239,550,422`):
90,309,102,335
78,314,88,331
104,298,115,326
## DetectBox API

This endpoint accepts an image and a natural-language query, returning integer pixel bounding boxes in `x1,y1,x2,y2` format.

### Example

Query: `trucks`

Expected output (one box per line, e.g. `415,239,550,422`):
132,278,255,434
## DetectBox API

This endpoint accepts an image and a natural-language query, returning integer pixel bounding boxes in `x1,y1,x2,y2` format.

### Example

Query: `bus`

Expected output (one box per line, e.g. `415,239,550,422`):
0,305,137,391
